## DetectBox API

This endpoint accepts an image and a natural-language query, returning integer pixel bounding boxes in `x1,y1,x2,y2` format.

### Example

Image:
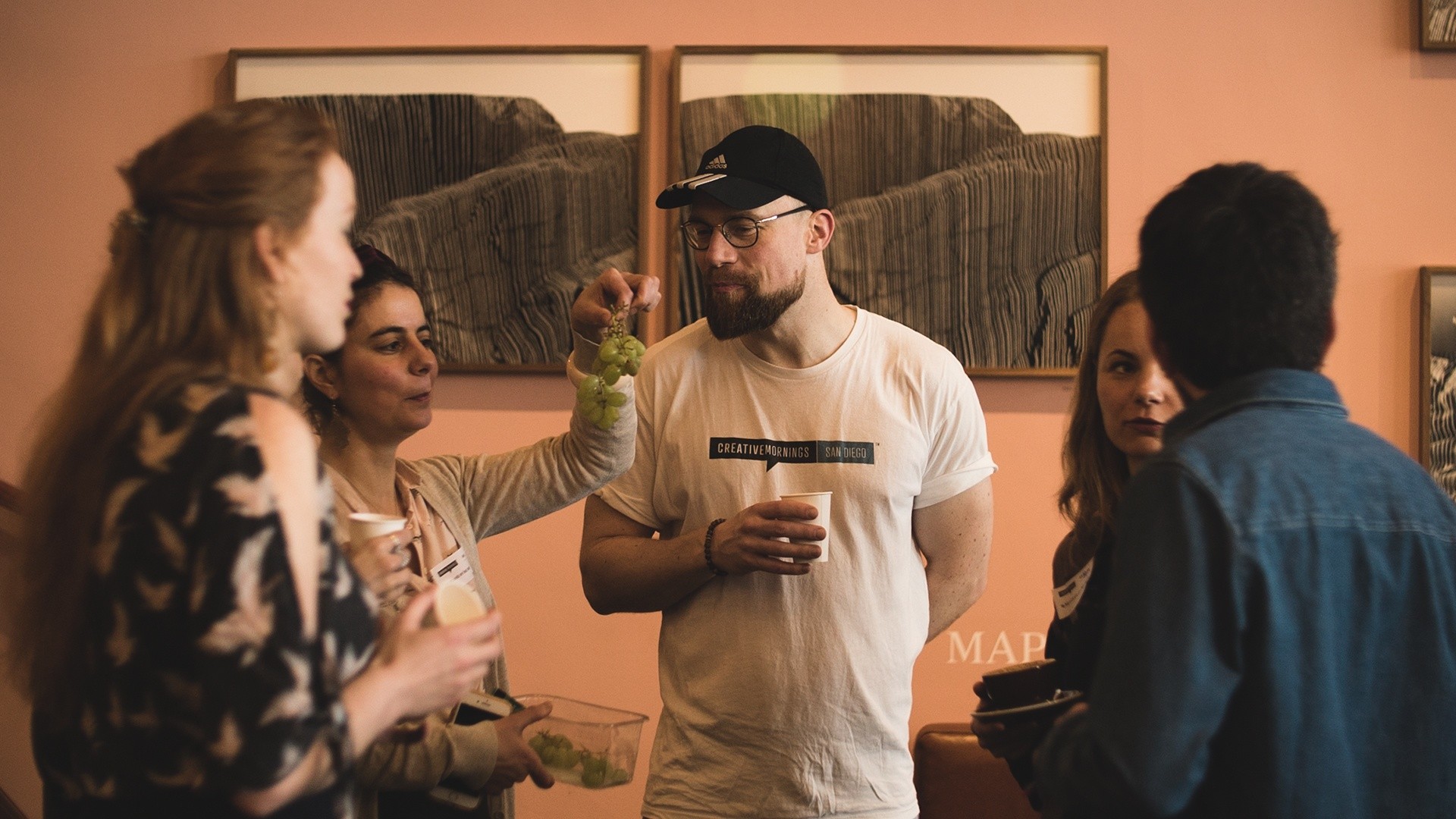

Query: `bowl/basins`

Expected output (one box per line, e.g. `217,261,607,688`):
514,693,650,790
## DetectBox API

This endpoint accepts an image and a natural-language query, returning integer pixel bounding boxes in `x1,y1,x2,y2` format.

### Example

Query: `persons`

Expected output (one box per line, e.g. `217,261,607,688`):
0,99,502,819
301,244,662,819
971,272,1186,812
579,126,996,819
1036,162,1456,819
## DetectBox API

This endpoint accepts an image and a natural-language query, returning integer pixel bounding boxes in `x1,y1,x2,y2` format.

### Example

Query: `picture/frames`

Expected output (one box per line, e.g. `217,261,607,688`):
225,46,651,377
1418,0,1456,51
1419,266,1456,503
669,44,1108,377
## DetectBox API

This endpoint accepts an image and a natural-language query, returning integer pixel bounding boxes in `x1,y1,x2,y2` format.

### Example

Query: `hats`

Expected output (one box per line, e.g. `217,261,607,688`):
655,126,828,215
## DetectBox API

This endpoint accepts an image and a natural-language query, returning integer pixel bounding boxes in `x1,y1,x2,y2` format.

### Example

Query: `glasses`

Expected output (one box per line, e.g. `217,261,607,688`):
679,205,816,251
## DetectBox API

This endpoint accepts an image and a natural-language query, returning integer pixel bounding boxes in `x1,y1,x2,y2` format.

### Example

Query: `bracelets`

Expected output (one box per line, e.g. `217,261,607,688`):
704,518,728,577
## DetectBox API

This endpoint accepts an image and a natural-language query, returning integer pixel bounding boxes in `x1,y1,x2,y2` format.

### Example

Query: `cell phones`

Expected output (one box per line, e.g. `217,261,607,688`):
424,690,513,811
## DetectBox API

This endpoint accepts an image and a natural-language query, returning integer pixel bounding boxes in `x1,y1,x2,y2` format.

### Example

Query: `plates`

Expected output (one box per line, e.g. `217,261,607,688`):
970,690,1085,723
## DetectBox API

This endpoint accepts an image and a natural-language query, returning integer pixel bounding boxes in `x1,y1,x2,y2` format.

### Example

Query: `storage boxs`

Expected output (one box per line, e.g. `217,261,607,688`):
514,693,650,789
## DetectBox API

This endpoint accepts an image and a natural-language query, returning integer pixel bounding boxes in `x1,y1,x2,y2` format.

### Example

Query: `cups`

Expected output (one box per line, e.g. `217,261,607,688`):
780,492,833,565
346,513,409,542
982,658,1055,709
